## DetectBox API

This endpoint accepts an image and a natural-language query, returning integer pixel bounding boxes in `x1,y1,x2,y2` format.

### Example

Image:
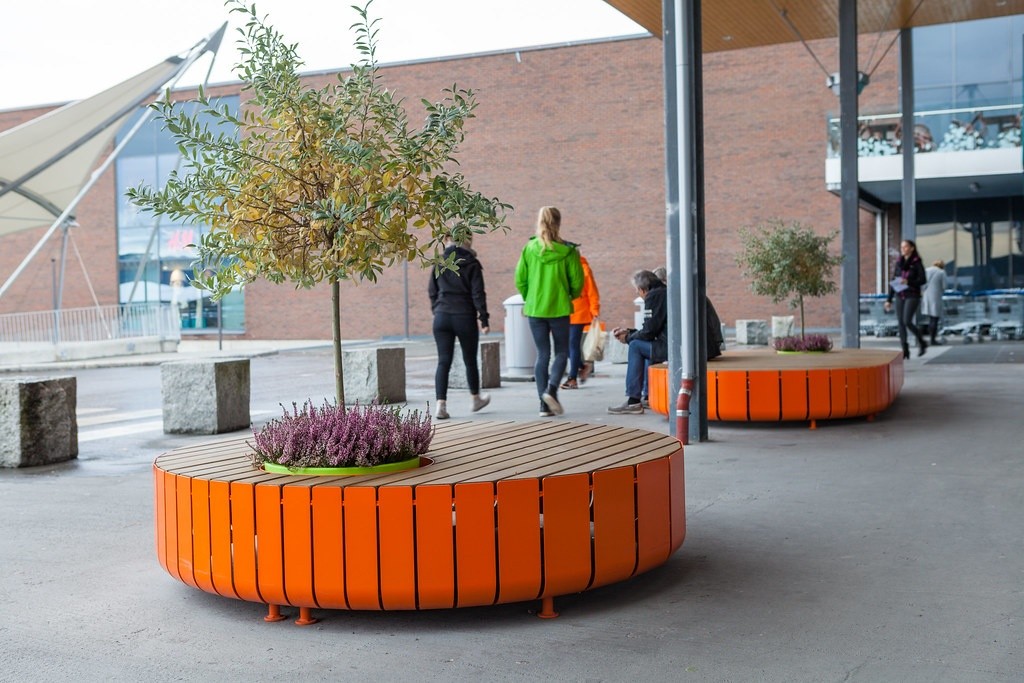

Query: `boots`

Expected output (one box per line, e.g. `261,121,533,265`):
901,341,910,360
917,338,928,356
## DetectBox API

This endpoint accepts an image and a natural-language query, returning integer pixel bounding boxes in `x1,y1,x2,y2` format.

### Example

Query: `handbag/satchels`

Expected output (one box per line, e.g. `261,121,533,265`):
582,317,606,363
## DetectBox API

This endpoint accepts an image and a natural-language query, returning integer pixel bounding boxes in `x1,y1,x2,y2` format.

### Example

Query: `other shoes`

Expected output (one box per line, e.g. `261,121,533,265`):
606,398,644,414
539,383,563,416
929,340,944,346
435,399,450,419
560,378,578,389
470,392,491,411
578,362,594,385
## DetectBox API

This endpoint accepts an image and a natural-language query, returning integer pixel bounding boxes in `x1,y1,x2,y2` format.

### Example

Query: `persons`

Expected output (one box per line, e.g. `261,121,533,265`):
563,247,600,389
608,270,721,412
514,206,585,416
921,260,949,346
429,224,490,418
886,240,927,360
652,266,723,351
